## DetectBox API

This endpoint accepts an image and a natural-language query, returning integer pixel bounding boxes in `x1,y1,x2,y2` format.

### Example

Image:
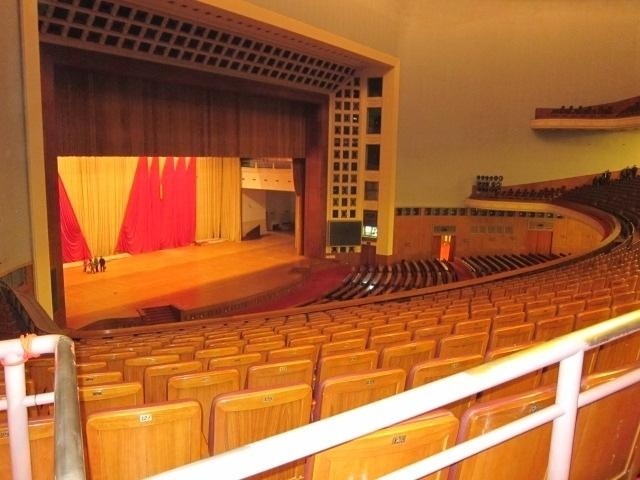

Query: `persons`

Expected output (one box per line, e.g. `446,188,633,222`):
94,256,99,272
88,258,95,273
98,256,105,272
82,257,87,273
